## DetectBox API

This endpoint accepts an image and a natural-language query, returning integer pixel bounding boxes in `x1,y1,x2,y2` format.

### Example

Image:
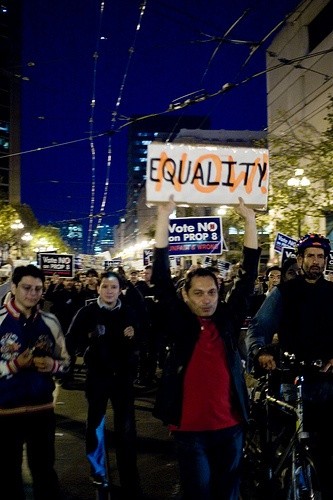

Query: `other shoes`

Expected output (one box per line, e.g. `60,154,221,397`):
88,466,110,488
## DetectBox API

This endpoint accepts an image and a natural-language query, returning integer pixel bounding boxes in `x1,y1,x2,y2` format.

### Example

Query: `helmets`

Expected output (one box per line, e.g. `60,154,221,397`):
298,234,331,255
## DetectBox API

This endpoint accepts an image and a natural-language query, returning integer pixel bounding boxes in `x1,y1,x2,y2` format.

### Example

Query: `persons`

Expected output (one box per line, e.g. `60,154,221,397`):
1,265,72,500
1,254,333,419
155,197,265,500
246,232,333,500
63,267,151,500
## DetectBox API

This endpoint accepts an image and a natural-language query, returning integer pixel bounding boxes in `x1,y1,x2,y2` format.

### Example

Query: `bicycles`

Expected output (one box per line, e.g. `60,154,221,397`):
246,350,333,500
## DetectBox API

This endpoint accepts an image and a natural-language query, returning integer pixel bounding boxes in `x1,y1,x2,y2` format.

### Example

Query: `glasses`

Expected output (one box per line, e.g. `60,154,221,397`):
16,285,45,293
